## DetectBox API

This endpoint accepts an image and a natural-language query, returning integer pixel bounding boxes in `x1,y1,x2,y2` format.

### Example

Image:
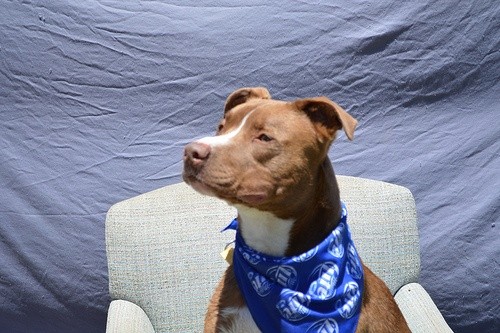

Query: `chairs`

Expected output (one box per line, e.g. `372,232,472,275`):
106,175,454,333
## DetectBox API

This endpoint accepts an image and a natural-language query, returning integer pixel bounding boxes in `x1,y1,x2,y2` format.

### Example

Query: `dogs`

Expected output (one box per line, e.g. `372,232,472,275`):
182,86,412,333
